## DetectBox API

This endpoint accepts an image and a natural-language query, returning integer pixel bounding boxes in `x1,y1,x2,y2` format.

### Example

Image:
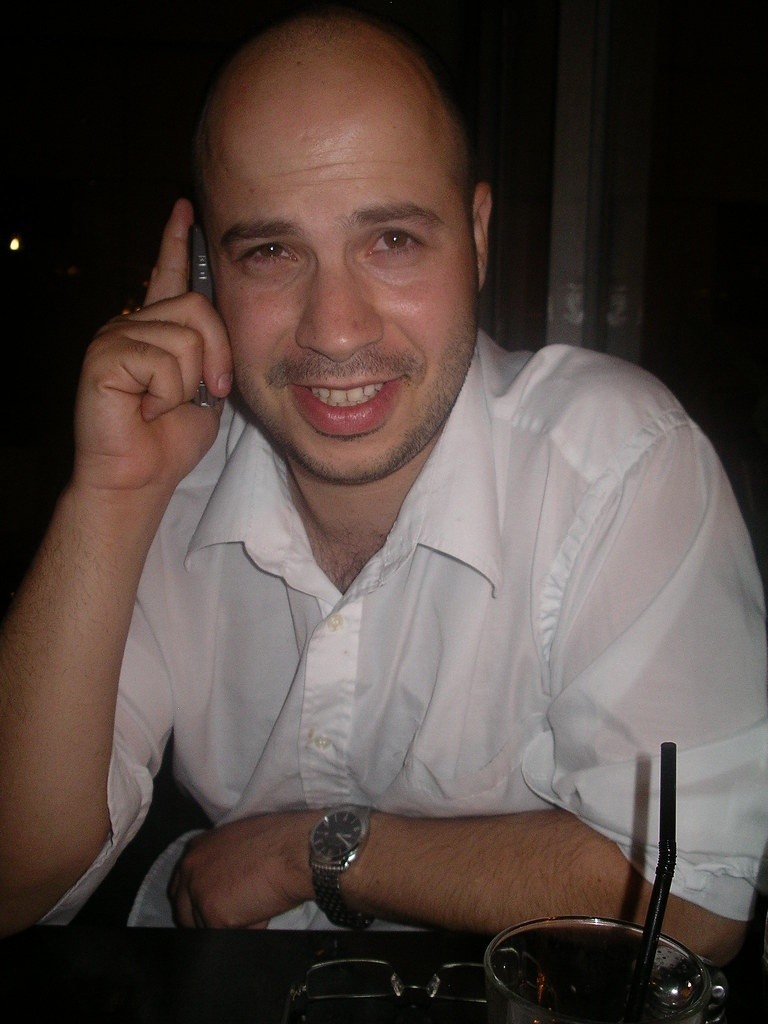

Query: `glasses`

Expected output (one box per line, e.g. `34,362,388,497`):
285,957,489,1024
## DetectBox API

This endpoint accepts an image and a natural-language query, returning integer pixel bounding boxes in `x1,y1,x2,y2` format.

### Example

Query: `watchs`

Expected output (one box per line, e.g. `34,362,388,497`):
309,804,371,930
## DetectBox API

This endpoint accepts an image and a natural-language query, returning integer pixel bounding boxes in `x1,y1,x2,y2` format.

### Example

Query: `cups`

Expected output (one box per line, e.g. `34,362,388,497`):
484,915,714,1024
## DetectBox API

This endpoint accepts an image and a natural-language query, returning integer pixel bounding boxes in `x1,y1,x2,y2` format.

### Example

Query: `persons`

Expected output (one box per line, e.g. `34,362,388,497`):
0,6,767,1024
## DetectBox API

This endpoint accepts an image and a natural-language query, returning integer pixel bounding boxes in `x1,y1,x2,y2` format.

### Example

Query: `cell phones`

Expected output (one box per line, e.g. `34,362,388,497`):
190,225,218,408
